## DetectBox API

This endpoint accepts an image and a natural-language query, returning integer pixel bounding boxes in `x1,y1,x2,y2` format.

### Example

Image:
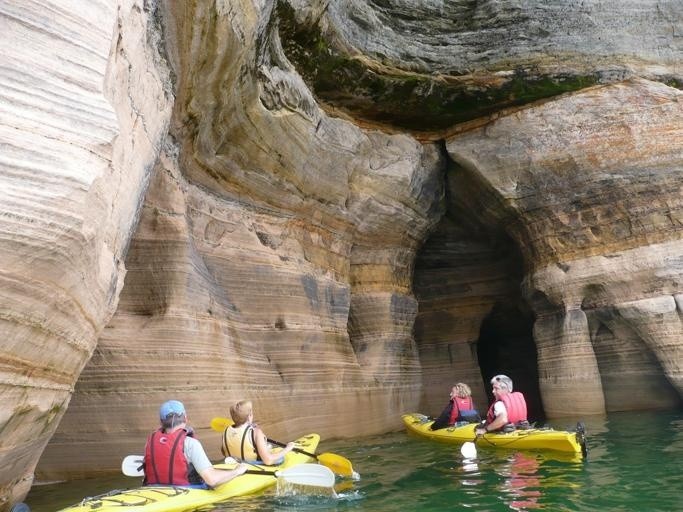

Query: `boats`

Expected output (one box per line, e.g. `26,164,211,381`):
61,434,320,512
404,414,584,456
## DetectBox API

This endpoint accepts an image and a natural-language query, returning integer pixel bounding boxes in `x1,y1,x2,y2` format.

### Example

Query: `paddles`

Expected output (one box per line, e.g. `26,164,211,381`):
121,455,336,488
461,423,486,458
209,417,354,478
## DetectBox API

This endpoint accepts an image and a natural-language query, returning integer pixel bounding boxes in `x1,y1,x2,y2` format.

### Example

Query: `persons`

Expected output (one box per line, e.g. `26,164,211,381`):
225,401,294,465
142,399,246,491
431,383,478,430
476,375,526,434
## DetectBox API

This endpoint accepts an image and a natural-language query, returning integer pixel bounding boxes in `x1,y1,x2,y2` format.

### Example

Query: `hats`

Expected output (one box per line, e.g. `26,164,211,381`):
491,375,513,393
161,400,185,422
